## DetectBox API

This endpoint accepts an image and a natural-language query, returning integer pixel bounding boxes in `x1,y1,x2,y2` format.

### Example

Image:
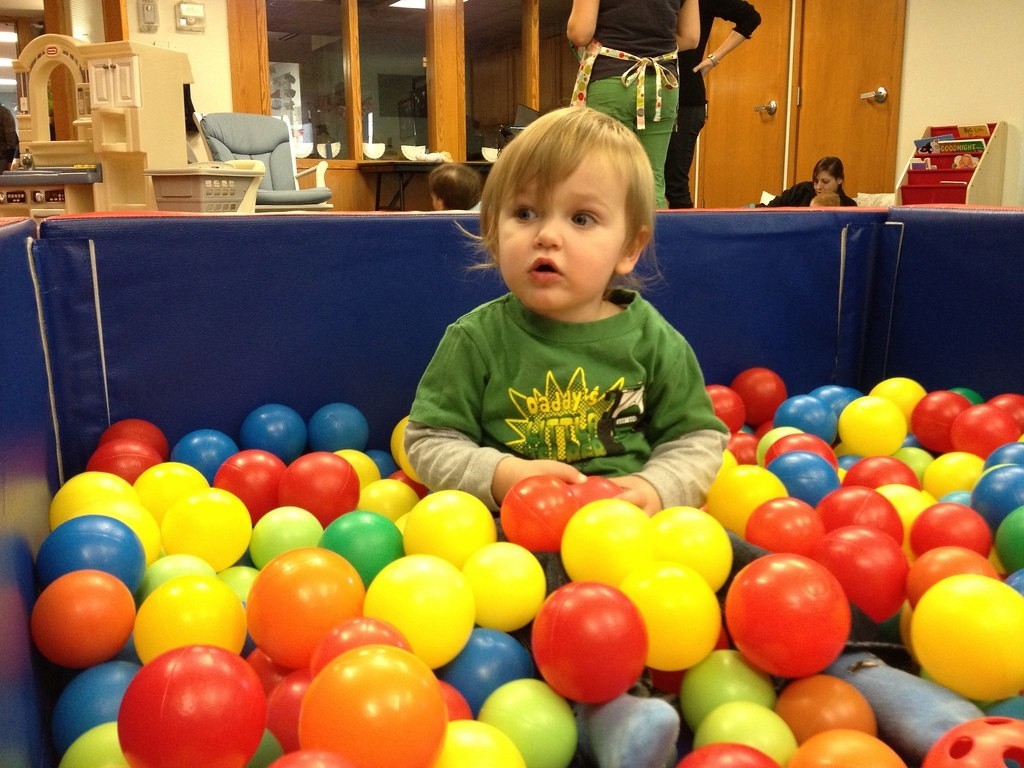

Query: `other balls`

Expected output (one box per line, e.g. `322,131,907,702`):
27,366,1024,768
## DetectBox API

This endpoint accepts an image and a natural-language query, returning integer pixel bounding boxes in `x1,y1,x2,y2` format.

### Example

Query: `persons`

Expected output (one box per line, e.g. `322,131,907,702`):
810,193,840,206
0,104,20,174
428,163,483,210
566,0,700,209
405,106,988,768
663,0,762,208
755,157,857,207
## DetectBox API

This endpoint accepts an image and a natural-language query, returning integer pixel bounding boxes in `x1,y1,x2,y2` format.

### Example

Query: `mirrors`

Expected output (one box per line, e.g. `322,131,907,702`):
47,64,78,141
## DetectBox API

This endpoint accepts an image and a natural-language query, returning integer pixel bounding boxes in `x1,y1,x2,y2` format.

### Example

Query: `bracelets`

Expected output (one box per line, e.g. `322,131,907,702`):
707,54,719,66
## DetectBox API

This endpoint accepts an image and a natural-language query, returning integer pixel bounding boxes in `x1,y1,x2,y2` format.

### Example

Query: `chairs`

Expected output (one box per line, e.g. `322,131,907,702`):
193,111,333,214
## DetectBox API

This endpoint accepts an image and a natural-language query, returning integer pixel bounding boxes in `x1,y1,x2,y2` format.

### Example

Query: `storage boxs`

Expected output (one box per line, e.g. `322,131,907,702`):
144,168,264,212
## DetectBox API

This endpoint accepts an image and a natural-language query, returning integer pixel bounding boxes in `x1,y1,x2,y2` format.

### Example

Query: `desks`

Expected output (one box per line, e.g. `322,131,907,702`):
357,159,495,210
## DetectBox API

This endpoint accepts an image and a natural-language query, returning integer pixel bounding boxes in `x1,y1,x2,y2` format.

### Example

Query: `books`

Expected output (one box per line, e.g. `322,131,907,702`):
909,124,990,184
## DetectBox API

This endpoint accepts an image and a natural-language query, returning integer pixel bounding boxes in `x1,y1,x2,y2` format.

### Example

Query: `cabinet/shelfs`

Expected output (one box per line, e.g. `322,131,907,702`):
895,120,1008,206
88,54,142,108
